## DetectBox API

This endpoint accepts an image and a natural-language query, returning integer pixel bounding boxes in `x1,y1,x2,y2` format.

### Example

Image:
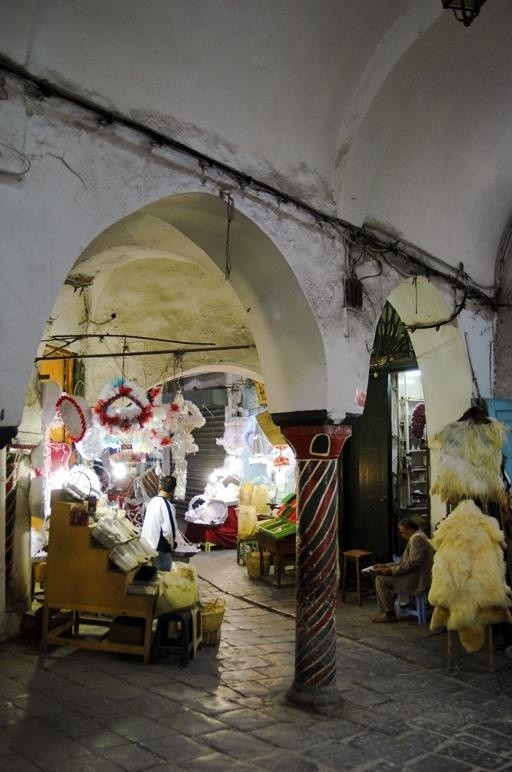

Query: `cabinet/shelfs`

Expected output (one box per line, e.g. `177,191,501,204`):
397,396,428,510
41,488,159,665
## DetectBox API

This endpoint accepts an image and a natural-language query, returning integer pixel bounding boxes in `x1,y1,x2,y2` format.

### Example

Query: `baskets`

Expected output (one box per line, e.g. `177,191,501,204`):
245,549,270,579
201,597,225,646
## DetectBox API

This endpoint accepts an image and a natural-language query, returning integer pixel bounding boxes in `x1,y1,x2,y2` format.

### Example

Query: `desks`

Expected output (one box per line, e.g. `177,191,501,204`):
256,531,295,588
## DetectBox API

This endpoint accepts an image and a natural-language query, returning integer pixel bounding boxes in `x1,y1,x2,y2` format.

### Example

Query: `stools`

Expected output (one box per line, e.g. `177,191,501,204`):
152,607,203,665
397,584,430,625
342,547,373,608
235,534,256,566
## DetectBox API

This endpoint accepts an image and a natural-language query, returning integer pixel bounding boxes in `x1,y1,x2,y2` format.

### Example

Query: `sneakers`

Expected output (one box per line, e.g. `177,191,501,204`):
372,612,397,623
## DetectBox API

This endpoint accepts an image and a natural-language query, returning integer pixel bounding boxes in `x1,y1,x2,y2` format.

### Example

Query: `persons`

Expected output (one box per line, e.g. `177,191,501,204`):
139,476,178,572
363,516,435,623
